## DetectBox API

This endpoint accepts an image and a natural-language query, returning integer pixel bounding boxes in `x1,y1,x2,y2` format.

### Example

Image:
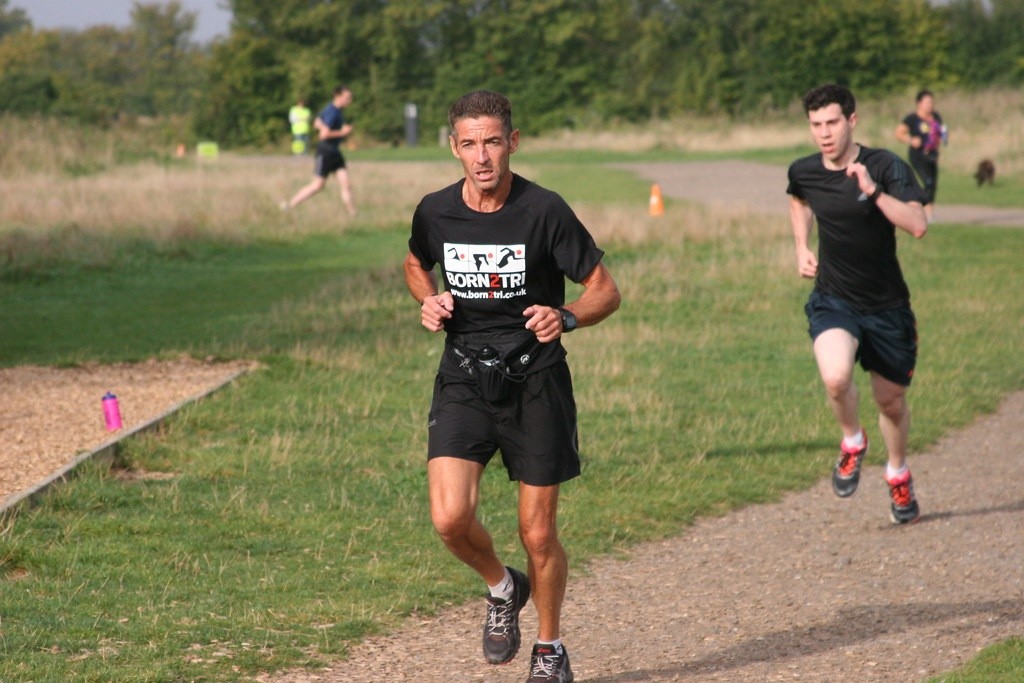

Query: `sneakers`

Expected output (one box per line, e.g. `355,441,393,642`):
882,469,921,525
830,429,868,499
484,565,531,665
524,644,574,683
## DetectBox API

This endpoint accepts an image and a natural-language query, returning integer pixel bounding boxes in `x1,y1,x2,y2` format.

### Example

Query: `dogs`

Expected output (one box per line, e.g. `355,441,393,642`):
973,160,995,188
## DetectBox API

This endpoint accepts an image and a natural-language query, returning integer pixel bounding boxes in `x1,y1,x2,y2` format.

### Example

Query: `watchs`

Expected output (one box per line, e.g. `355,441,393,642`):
555,307,577,333
867,184,885,201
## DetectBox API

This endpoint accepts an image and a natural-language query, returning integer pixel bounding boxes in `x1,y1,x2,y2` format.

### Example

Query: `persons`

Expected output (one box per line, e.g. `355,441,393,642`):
402,90,622,683
279,87,358,216
898,90,948,222
288,100,311,155
785,84,930,524
